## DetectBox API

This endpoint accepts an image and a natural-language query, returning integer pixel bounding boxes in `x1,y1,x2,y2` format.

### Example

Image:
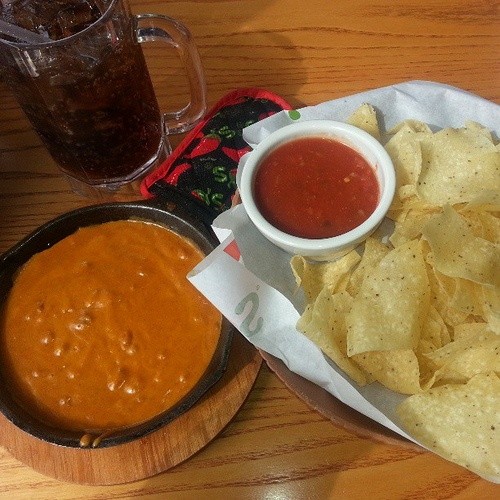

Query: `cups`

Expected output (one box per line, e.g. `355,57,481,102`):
0,0,205,201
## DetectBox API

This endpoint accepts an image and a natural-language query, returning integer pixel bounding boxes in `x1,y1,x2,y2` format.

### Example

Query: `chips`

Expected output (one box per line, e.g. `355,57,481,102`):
290,104,500,486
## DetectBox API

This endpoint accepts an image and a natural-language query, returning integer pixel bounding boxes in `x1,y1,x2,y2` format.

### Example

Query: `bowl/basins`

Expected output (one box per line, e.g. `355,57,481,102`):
258,350,422,455
0,204,230,450
242,119,397,267
